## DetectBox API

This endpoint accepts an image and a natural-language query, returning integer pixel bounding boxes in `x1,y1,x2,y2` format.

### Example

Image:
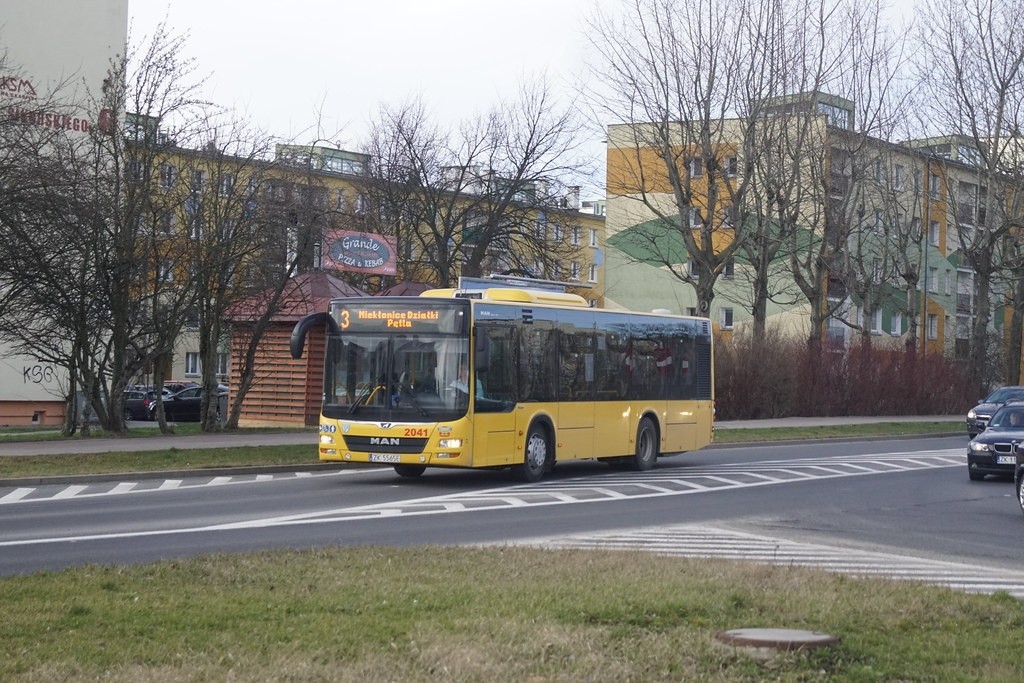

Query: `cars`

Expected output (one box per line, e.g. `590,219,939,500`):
118,378,229,423
967,385,1024,437
966,398,1024,482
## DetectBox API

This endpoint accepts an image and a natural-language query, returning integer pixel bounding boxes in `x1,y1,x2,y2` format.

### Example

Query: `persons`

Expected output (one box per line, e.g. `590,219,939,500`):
1009,412,1020,426
450,360,484,400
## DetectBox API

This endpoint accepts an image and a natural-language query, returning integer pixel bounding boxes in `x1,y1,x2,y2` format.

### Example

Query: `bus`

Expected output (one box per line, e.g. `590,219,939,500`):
289,274,717,483
